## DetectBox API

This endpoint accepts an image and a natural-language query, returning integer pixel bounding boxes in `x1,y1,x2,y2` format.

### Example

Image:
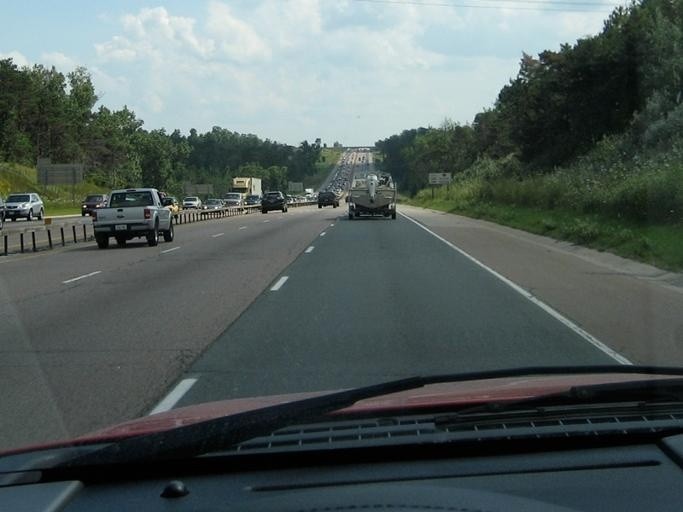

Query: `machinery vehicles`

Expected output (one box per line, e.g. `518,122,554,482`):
344,171,398,220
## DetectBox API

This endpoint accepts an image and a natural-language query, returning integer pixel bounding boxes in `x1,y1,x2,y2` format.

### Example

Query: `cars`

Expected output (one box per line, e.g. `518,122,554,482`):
80,192,261,217
286,148,371,205
0,197,6,230
3,192,45,222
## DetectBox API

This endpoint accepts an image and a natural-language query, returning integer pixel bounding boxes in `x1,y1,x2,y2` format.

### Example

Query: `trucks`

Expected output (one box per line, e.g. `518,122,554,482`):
231,176,262,199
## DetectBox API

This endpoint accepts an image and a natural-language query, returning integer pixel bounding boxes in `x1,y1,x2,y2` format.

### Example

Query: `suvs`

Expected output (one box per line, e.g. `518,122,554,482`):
260,190,289,214
317,191,340,209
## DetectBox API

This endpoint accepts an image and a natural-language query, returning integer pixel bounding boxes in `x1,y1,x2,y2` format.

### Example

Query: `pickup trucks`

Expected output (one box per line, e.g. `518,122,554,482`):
91,187,176,250
